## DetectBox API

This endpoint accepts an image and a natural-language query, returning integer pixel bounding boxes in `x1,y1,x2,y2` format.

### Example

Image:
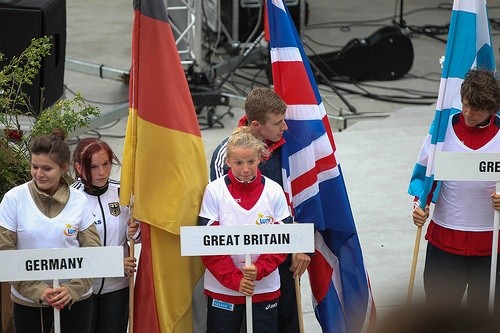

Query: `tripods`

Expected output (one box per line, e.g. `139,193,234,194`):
393,0,447,44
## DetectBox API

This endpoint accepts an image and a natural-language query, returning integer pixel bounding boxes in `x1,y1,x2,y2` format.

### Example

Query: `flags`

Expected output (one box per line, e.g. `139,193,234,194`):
265,0,377,333
408,0,496,212
120,0,209,333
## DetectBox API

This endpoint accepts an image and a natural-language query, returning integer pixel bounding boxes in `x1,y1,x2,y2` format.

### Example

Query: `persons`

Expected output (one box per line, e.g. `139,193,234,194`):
70,138,142,333
412,67,500,307
0,128,102,333
211,87,311,333
198,125,292,333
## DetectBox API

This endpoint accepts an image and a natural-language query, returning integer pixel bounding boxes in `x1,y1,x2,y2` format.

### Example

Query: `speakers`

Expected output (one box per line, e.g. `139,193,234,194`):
0,0,66,113
